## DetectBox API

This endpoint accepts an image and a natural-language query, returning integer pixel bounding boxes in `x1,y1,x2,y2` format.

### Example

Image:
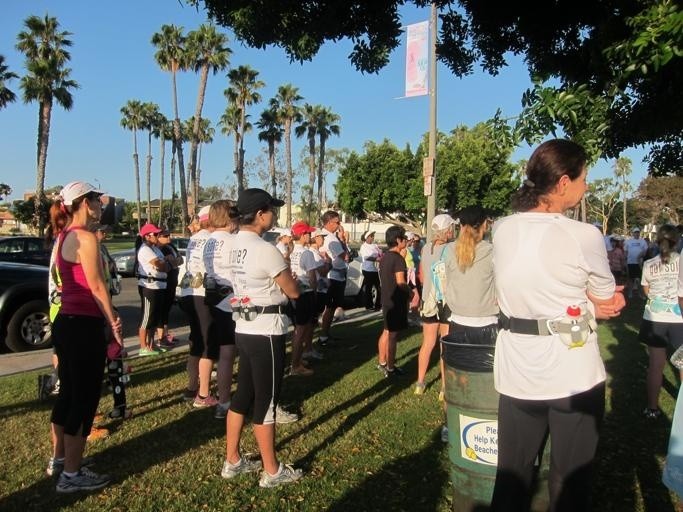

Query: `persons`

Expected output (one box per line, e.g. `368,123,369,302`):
492,139,628,511
640,225,683,421
592,222,658,298
662,250,683,499
48,181,501,493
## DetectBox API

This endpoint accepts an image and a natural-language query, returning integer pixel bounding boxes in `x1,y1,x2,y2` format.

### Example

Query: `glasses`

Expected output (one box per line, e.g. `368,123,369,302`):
96,223,109,231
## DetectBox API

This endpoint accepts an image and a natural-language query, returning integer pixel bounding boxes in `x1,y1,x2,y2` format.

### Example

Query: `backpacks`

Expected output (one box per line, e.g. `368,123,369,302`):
430,259,447,307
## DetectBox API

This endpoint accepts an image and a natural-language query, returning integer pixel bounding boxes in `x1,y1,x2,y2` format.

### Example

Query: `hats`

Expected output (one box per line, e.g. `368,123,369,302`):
236,188,285,215
289,222,317,235
458,205,487,228
138,224,161,238
430,213,458,230
196,205,210,222
310,227,327,238
279,229,291,237
364,230,376,240
632,227,639,233
57,181,106,207
404,231,415,240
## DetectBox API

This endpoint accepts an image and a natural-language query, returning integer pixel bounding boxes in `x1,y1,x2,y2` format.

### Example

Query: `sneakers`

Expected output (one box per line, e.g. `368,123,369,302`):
84,426,110,441
137,345,159,356
288,366,314,377
258,461,304,488
93,410,104,424
316,336,341,347
302,349,324,360
385,367,409,380
156,338,175,348
46,455,96,476
219,456,264,480
377,363,387,372
213,404,231,419
639,406,662,421
150,344,167,353
192,393,219,408
164,334,179,345
275,405,298,424
56,468,112,492
438,389,445,402
413,383,427,395
37,373,55,402
183,387,211,400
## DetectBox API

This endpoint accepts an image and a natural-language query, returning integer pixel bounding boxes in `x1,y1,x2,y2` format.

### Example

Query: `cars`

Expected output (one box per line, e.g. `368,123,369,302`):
0,235,122,300
261,227,383,309
0,261,51,352
110,236,189,277
